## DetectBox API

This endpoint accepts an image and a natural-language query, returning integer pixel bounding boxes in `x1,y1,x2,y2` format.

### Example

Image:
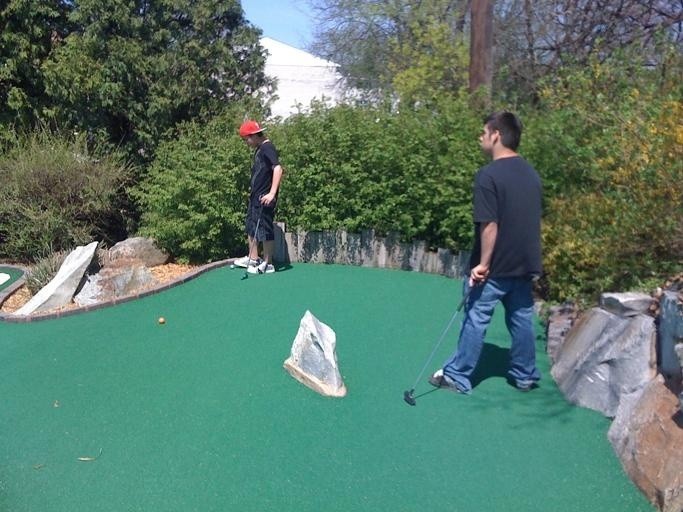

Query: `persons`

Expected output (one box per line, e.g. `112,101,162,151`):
429,111,541,394
234,120,283,275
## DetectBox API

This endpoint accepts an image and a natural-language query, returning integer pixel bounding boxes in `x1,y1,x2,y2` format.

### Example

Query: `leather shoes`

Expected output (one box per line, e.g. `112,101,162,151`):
429,369,459,392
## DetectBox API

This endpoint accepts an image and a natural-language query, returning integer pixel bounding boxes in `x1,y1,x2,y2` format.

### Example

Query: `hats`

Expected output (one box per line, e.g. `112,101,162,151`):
240,121,266,136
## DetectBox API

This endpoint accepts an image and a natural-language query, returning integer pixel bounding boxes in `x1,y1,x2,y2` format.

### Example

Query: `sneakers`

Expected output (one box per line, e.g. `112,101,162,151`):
234,256,275,274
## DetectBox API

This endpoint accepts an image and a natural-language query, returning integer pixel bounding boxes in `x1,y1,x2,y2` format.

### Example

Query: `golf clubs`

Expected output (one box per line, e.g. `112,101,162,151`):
242,198,264,281
404,276,484,406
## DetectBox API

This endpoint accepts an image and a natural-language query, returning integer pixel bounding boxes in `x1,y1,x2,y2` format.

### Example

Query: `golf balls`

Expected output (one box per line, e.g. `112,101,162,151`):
158,317,165,324
230,265,234,268
